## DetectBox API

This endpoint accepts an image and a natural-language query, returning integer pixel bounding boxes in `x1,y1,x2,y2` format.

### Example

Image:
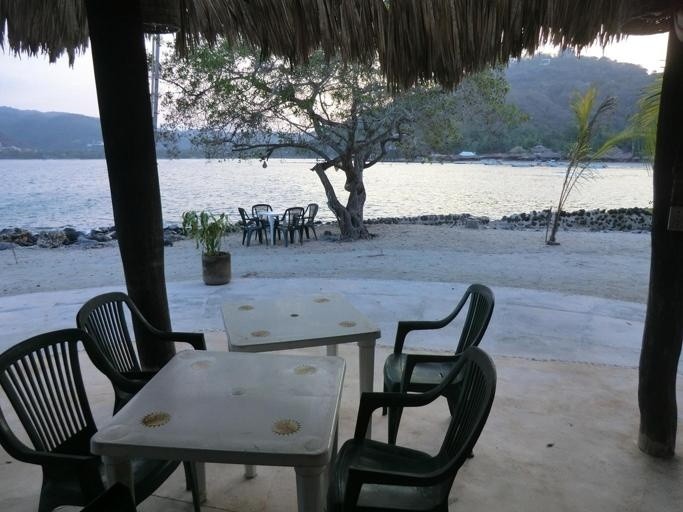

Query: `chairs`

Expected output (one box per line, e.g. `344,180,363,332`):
0,327,207,511
237,203,319,248
75,291,207,496
328,282,497,512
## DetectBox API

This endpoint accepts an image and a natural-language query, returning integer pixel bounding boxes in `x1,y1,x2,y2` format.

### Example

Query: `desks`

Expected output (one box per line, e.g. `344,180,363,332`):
214,295,381,481
84,346,348,511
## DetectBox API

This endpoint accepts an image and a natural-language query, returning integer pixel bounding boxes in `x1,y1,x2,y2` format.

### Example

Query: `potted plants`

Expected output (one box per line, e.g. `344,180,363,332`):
182,208,232,285
